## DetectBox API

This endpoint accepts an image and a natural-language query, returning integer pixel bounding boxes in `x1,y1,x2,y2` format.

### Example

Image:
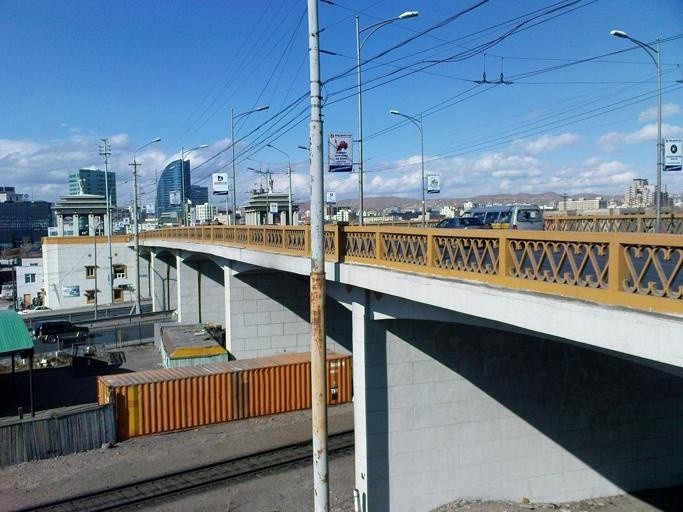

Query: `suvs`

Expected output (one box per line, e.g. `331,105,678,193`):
32,320,90,344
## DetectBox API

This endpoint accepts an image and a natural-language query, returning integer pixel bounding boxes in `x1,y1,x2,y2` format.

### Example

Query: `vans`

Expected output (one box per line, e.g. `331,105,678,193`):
460,203,545,230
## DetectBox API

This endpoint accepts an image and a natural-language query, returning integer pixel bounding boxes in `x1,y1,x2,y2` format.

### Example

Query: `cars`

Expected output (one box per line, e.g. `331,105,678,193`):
22,306,53,314
436,217,492,230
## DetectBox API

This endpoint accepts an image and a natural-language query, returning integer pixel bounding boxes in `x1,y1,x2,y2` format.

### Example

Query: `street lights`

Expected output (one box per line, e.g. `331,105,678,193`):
181,145,208,225
109,181,127,235
611,29,662,232
231,106,293,226
354,10,428,227
85,222,105,319
133,138,161,313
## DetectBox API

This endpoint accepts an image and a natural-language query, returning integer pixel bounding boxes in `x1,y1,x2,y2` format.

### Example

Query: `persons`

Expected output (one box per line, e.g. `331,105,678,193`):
32,296,42,306
